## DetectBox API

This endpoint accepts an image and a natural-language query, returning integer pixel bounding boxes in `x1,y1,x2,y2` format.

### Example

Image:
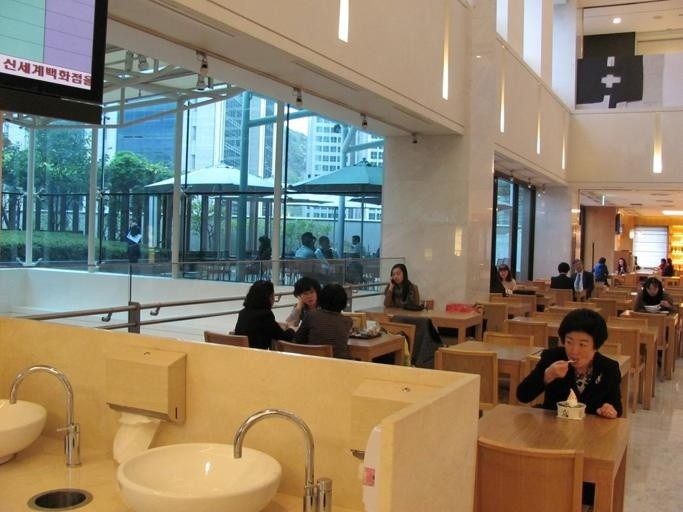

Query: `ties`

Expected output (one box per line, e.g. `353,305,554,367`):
575,274,580,291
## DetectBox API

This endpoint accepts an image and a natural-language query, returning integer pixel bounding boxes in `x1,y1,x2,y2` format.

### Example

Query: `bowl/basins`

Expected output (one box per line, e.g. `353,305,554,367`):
643,304,661,314
556,401,586,419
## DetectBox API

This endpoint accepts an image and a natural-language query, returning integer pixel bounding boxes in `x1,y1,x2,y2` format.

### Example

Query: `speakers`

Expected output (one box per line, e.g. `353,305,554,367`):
583,32,635,57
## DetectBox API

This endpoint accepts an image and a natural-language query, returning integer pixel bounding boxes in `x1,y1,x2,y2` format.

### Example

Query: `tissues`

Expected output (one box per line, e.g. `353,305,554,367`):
103,344,187,466
349,376,446,481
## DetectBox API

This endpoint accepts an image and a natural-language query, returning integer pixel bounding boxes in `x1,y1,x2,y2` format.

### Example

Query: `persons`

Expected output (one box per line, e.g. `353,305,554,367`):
633,277,673,368
497,265,517,291
550,256,675,301
127,219,141,263
296,284,353,360
346,261,367,290
516,308,622,419
314,236,342,273
348,236,371,258
247,235,272,274
384,264,419,307
234,280,299,350
285,276,322,327
295,232,328,280
491,264,506,297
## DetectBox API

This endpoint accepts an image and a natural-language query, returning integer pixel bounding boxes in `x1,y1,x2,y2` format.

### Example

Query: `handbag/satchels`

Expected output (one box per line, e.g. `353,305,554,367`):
403,303,428,312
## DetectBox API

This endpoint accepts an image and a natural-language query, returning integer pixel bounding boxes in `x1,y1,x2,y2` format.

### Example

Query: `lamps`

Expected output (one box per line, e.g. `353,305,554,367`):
194,50,419,145
507,170,546,195
136,53,148,70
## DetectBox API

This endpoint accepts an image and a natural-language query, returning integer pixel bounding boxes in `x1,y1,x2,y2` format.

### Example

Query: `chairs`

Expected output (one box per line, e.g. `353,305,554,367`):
177,251,682,512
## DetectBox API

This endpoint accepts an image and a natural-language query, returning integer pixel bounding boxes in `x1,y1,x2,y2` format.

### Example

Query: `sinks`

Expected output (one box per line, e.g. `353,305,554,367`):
116,442,283,512
0,399,47,464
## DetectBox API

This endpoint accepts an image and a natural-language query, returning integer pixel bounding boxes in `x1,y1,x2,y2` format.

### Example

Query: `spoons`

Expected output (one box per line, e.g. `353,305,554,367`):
567,359,576,363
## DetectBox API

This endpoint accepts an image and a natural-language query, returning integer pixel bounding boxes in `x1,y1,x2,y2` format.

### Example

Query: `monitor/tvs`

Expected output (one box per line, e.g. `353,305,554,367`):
1,0,108,125
616,213,622,233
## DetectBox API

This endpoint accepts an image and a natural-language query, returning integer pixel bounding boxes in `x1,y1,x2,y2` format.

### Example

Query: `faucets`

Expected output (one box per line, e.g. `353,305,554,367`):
233,408,332,512
9,364,82,468
